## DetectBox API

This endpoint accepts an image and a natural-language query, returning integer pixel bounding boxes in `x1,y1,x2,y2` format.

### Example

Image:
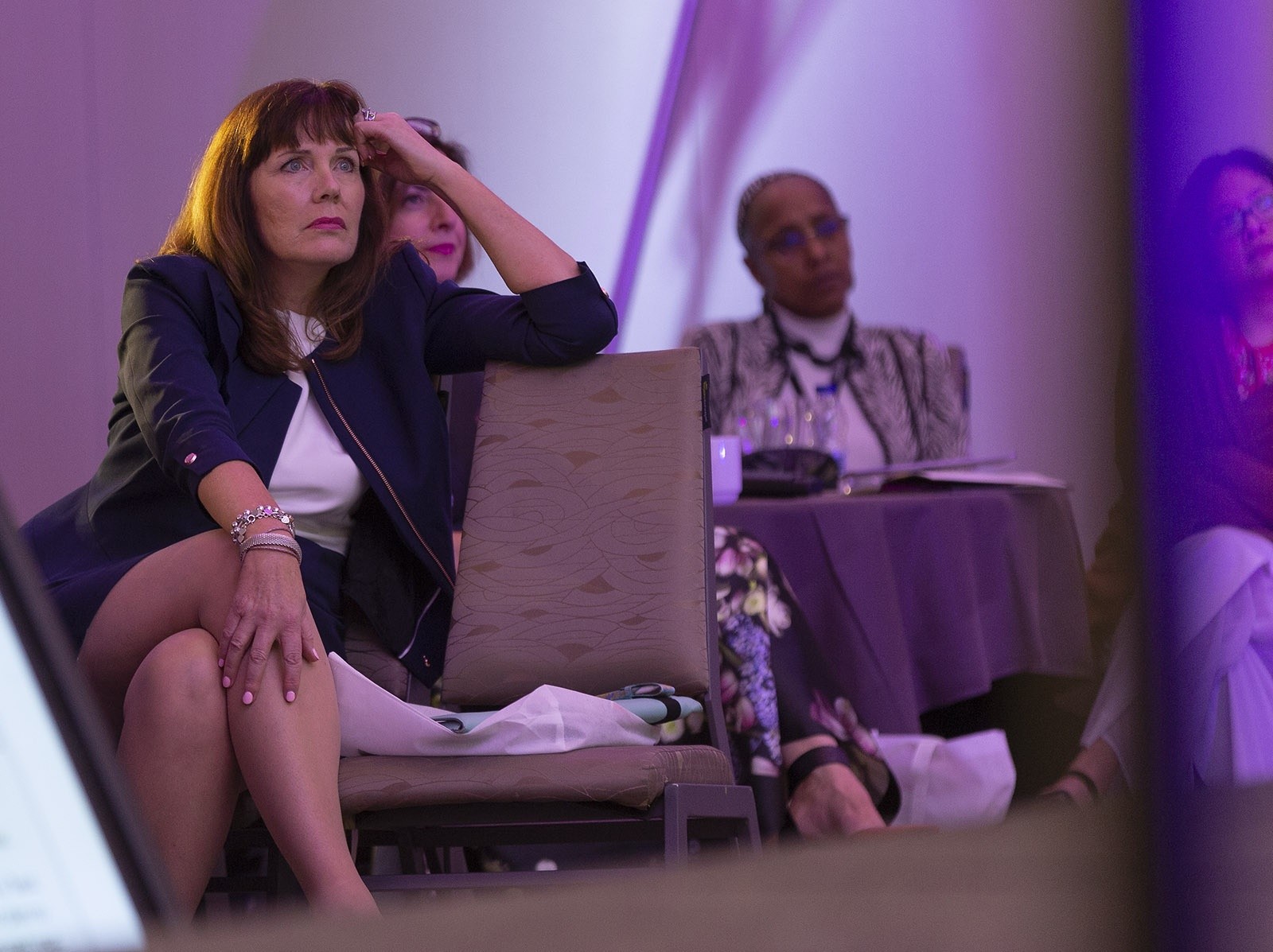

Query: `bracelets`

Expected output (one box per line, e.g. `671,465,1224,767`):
361,107,377,121
228,504,303,545
239,527,303,567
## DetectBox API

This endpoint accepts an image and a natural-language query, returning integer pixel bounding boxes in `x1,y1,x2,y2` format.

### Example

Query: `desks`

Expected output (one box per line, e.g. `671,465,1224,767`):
711,482,1097,743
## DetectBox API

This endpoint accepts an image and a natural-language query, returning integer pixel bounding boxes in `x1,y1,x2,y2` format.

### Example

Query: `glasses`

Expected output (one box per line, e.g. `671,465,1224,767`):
749,216,846,258
1210,193,1273,238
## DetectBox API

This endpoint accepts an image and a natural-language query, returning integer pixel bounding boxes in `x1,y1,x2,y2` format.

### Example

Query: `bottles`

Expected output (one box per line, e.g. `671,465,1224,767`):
806,369,854,499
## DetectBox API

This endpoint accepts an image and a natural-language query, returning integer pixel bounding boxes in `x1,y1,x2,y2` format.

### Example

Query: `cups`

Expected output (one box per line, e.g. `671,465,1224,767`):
709,435,744,506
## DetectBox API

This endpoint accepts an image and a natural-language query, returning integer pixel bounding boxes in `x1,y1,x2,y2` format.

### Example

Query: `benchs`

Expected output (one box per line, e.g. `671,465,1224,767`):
222,344,760,861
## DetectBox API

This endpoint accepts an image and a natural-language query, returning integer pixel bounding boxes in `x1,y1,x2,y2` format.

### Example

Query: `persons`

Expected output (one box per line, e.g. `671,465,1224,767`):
1004,145,1273,817
46,78,980,940
685,168,975,492
